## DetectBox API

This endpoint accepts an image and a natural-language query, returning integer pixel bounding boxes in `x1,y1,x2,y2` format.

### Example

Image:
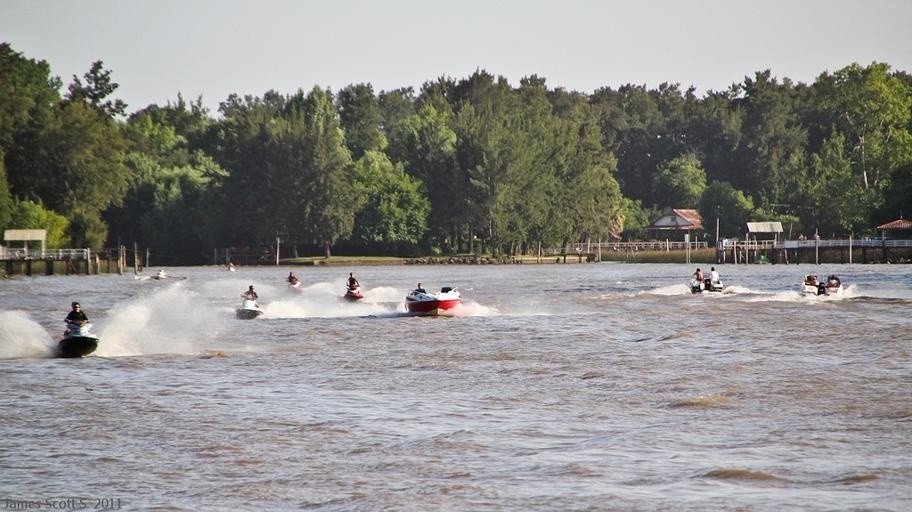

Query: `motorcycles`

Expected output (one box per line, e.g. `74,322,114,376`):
58,323,99,359
234,295,264,320
800,273,846,298
289,280,304,294
345,285,362,301
690,278,722,293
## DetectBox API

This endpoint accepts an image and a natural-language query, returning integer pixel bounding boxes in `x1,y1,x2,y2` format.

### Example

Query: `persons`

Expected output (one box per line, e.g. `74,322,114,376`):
414,283,427,297
290,275,300,286
346,272,360,293
694,267,704,284
244,284,258,300
288,272,294,281
241,296,260,309
157,268,166,276
707,266,720,283
228,262,235,270
63,301,92,336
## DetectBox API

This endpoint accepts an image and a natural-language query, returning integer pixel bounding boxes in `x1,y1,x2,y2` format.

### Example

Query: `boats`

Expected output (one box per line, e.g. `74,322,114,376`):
135,269,189,281
225,265,237,272
405,287,463,316
